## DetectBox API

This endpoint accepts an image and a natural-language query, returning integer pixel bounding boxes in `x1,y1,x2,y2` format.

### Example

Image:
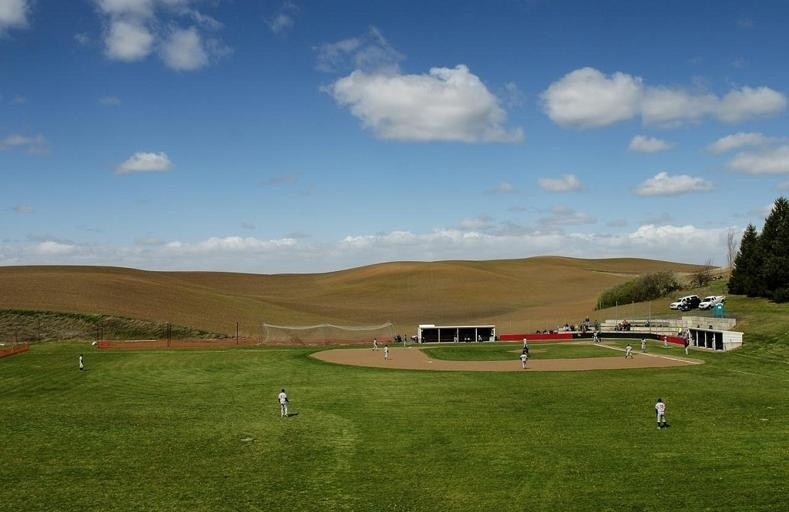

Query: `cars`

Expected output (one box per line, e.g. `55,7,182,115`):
394,335,402,342
411,335,419,342
680,298,701,312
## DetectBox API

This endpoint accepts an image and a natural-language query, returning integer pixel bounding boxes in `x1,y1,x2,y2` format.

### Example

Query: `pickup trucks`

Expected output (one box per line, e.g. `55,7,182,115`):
698,295,726,311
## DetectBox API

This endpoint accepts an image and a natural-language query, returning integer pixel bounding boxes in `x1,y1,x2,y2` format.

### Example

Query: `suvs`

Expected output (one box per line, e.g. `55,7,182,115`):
670,295,700,310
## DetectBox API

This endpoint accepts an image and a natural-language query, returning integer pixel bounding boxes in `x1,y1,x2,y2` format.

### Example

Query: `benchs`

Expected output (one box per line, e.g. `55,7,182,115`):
587,319,685,334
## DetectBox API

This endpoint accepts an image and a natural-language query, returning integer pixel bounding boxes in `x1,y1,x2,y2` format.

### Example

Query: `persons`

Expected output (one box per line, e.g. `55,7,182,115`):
78,353,85,371
535,315,650,343
520,350,529,369
663,335,668,346
392,333,484,344
641,338,647,352
685,336,696,355
372,338,378,351
277,389,291,418
654,398,668,430
520,338,528,359
624,343,633,360
382,344,390,360
676,328,691,348
